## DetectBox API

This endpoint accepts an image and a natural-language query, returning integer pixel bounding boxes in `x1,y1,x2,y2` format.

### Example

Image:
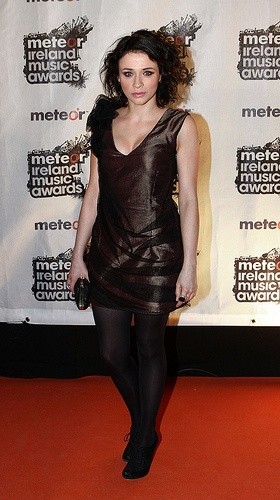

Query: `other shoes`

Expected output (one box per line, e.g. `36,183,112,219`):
121,431,159,480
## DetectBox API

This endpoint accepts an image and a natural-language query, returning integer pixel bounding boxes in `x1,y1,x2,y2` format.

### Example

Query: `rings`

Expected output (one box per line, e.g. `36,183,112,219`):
178,295,186,303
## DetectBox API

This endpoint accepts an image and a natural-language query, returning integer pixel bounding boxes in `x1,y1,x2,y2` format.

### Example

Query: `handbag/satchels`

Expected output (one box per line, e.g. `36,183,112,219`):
73,242,90,310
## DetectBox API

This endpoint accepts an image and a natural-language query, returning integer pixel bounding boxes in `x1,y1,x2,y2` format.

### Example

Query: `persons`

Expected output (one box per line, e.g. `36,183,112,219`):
68,29,199,478
